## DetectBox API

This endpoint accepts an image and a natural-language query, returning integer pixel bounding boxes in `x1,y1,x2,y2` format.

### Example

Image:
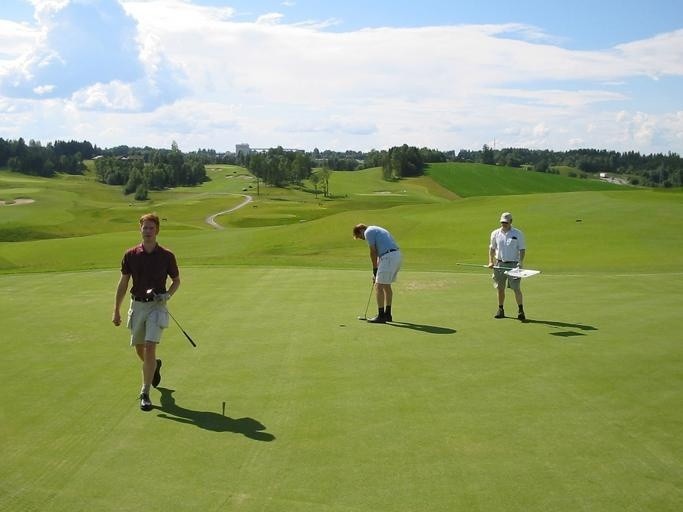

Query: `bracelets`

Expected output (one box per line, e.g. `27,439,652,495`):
372,268,378,276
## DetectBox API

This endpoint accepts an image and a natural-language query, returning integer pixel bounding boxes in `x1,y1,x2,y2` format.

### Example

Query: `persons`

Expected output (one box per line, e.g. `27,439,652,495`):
487,211,528,319
112,212,179,413
348,221,402,325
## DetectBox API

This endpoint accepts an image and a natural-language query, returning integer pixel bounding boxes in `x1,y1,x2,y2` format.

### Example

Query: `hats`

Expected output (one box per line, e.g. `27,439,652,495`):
500,213,512,222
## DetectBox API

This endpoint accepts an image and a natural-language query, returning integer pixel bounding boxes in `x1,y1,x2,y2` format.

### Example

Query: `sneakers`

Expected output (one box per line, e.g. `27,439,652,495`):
518,311,525,320
495,309,504,317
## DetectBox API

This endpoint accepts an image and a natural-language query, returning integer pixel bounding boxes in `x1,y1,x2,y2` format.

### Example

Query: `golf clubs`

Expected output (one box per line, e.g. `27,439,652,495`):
359,272,376,320
146,288,197,347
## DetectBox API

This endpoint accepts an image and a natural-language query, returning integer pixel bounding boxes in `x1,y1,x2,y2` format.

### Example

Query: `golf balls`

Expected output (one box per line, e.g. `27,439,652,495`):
358,316,360,319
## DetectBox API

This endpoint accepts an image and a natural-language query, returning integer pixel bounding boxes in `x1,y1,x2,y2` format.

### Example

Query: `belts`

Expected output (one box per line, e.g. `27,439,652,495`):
498,260,518,263
382,248,396,256
131,295,154,302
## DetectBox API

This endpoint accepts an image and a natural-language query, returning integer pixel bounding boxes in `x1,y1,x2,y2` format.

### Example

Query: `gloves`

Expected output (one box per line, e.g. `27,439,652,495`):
154,293,170,304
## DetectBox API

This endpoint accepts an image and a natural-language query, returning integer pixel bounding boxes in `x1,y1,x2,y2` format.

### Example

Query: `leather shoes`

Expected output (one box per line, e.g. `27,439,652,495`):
152,359,161,387
139,392,152,411
368,314,392,323
